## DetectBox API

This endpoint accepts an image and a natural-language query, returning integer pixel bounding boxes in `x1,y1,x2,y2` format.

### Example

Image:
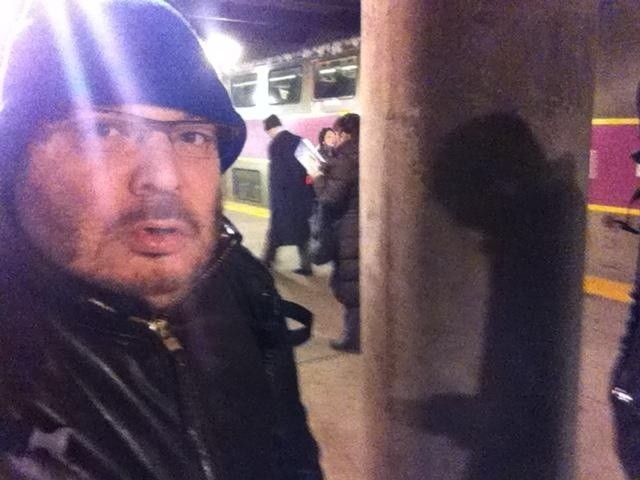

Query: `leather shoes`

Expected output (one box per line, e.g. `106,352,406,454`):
291,266,314,278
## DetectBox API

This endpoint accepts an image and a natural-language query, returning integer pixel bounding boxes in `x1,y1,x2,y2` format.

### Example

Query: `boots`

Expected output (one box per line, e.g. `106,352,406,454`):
328,308,361,355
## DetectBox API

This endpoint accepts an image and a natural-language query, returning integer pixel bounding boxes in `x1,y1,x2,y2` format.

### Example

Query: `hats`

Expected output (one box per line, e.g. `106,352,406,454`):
263,115,281,132
0,0,248,175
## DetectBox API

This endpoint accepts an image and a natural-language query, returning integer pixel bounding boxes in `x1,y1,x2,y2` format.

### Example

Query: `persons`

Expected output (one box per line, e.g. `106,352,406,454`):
317,127,334,161
2,2,323,480
260,111,316,275
375,108,591,480
317,109,362,352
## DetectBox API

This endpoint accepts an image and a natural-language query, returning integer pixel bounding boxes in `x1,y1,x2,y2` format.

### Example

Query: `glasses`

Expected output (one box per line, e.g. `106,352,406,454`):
60,104,241,160
